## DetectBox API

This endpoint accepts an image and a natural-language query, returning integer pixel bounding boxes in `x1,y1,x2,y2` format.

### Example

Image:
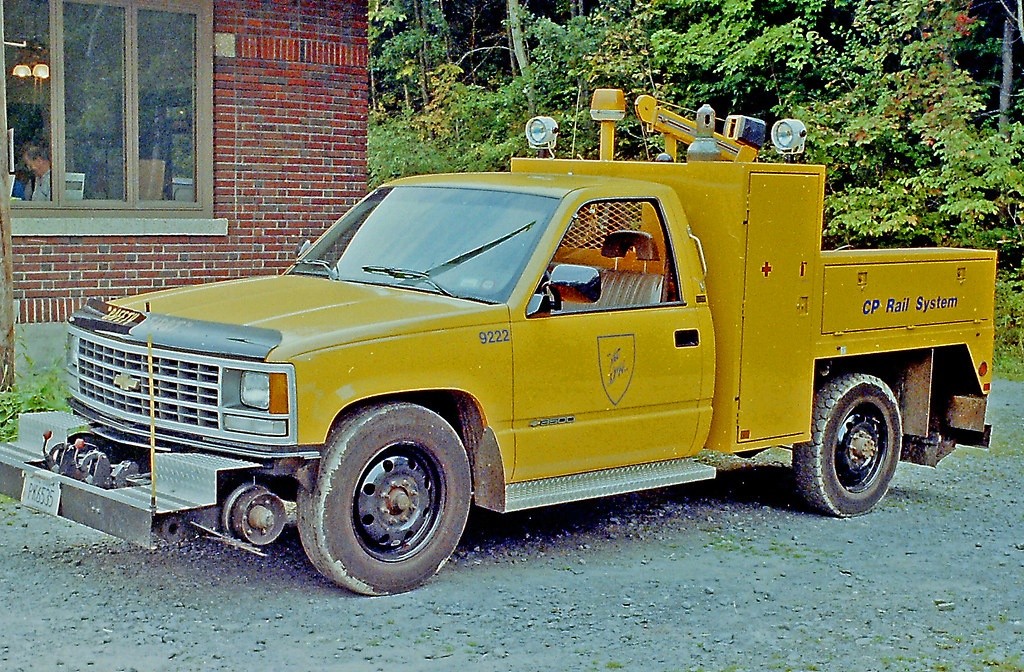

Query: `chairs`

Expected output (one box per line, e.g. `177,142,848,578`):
553,231,664,315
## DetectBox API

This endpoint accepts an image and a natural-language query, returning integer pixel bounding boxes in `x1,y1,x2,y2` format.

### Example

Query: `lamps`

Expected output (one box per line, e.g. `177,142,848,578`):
11,41,51,81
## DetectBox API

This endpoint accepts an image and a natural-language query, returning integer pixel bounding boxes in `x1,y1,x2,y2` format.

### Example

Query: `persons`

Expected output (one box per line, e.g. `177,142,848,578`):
10,142,52,201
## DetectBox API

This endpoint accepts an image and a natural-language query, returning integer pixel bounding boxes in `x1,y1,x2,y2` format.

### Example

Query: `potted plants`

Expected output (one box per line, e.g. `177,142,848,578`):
77,49,193,201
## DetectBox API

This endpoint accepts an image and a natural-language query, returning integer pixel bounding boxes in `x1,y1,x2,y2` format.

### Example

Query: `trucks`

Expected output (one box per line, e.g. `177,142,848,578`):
0,87,1000,599
